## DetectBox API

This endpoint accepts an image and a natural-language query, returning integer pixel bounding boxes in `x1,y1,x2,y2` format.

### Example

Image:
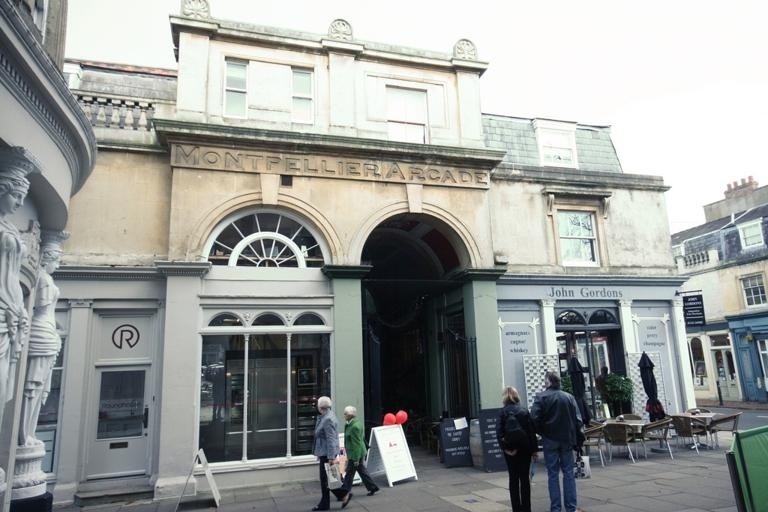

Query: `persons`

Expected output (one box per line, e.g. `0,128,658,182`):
382,412,396,425
340,405,384,496
395,409,407,424
530,371,587,511
310,395,352,511
1,173,29,482
494,384,536,511
595,365,609,403
22,250,63,450
212,367,226,421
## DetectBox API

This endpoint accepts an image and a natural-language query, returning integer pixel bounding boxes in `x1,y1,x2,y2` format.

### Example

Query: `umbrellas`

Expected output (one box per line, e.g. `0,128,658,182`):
567,356,591,424
638,351,666,421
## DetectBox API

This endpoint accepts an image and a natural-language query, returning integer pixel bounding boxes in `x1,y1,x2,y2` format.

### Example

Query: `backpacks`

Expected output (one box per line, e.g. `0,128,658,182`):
498,407,531,454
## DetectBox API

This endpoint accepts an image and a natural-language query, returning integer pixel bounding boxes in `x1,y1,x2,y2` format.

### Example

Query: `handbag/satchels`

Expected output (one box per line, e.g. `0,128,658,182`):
572,446,591,480
528,455,537,479
324,461,342,490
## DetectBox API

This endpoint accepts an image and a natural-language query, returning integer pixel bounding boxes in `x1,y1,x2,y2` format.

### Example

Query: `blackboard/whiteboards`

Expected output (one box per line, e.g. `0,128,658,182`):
440,416,474,467
373,423,416,483
478,407,509,472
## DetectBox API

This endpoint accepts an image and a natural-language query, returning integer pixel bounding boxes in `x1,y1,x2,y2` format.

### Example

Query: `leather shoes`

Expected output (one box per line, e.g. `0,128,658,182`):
312,505,329,511
367,487,379,497
340,493,354,509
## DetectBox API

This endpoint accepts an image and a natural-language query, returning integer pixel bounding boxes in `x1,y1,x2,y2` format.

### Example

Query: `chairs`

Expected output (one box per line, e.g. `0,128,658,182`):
405,415,442,459
578,406,743,469
